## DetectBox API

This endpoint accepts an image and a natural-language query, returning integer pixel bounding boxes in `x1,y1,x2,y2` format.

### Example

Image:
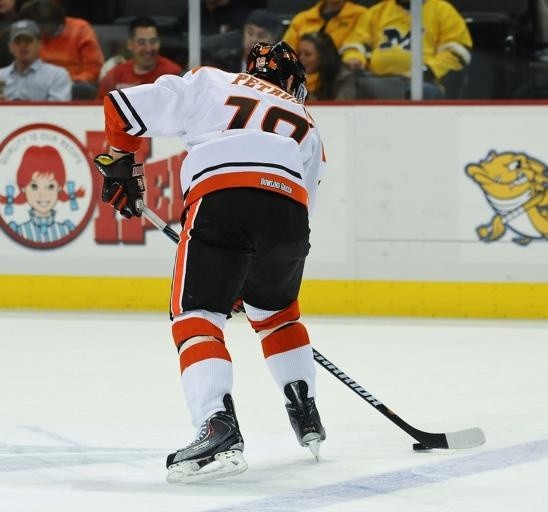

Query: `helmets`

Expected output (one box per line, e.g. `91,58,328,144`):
246,40,305,83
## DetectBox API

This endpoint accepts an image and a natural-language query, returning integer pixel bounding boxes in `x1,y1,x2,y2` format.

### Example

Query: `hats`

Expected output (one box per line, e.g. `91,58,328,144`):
10,20,41,45
245,10,279,33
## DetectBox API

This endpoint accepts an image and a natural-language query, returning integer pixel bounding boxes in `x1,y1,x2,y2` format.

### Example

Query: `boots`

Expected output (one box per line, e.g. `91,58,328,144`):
284,379,326,448
166,394,243,468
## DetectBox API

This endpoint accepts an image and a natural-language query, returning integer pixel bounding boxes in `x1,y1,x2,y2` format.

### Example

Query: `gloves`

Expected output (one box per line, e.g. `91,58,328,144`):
94,154,144,220
231,297,243,313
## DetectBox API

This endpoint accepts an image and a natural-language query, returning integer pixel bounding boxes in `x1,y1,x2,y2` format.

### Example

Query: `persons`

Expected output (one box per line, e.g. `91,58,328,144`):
93,41,326,472
201,0,473,101
0,146,84,242
0,0,186,101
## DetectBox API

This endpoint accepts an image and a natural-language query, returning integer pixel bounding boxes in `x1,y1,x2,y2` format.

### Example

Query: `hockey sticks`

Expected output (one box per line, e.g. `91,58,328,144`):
142,206,485,450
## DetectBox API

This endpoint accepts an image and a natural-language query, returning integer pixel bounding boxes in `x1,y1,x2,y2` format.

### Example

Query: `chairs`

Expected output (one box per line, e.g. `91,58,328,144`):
0,0,548,103
